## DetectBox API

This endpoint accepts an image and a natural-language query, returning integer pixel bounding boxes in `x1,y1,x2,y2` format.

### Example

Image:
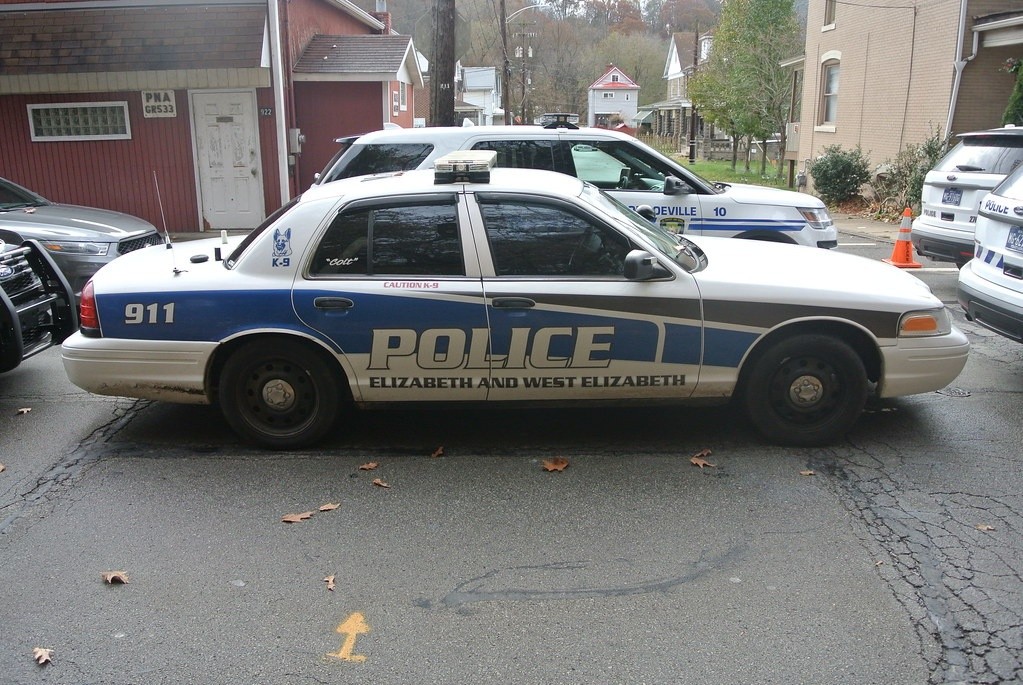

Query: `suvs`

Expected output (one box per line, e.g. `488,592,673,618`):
908,123,1023,268
307,118,840,251
959,161,1023,344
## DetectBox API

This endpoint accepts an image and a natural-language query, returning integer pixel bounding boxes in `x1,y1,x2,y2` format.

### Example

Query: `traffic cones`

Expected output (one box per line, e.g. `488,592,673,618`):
882,207,923,269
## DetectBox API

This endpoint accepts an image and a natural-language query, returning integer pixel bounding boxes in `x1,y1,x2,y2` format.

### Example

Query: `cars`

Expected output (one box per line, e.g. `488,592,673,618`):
62,147,973,451
1,176,165,311
